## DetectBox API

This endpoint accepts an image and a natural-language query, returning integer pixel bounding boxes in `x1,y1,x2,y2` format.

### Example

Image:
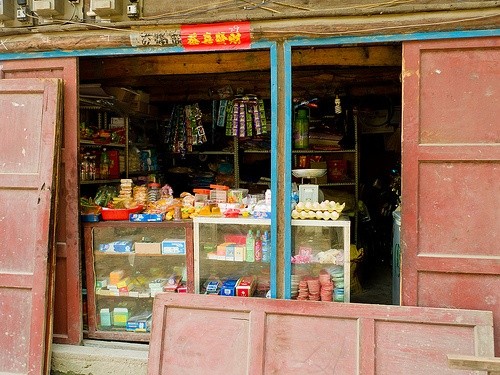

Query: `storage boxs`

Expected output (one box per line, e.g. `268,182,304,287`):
99,307,152,332
221,274,257,297
113,237,187,256
106,87,150,111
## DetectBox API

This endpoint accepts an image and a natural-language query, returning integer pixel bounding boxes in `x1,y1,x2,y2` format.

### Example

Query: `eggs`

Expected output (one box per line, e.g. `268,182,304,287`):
293,200,338,220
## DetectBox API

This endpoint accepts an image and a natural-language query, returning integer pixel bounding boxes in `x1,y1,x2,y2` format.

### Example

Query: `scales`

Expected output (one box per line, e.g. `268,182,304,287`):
291,169,327,203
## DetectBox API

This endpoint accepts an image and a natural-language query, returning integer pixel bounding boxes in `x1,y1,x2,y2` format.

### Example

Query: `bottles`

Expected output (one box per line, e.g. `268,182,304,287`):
133,180,147,204
255,230,262,262
262,231,270,263
147,183,161,203
251,189,271,204
245,230,255,262
81,150,97,181
293,105,310,148
100,148,110,180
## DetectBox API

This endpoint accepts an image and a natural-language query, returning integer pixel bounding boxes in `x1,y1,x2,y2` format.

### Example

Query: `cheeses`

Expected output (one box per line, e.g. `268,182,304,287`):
198,206,221,215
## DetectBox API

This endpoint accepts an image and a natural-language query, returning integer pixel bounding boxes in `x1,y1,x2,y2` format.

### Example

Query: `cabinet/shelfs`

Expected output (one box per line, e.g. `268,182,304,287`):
194,215,354,303
77,103,168,185
83,213,192,343
169,103,275,191
291,105,361,243
